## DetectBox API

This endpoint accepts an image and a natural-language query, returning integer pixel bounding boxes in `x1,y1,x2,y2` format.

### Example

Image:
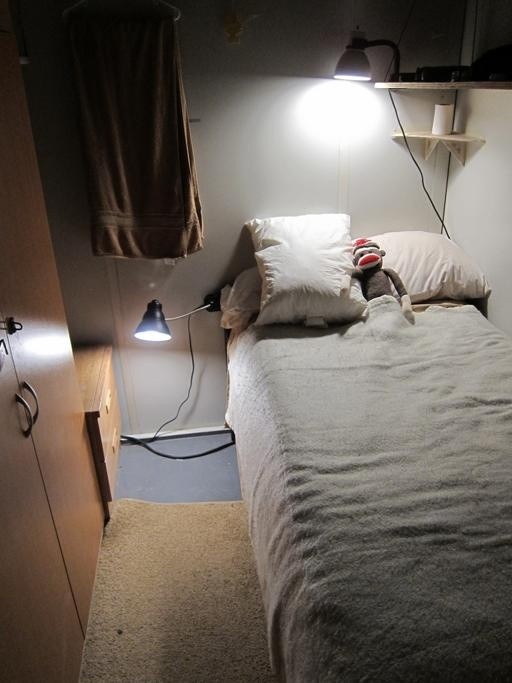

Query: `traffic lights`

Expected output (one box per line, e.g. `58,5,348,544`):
245,212,366,326
224,231,492,331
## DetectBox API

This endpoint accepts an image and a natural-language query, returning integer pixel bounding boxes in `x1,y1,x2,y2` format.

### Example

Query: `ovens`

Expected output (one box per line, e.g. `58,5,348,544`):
221,279,512,683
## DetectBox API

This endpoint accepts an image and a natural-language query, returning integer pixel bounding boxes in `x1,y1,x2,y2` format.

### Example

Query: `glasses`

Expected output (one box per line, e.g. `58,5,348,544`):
333,26,401,81
134,292,220,342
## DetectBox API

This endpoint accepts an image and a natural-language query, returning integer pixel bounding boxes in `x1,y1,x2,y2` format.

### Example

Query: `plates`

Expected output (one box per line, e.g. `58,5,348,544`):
0,1,106,683
374,81,512,166
72,343,119,518
